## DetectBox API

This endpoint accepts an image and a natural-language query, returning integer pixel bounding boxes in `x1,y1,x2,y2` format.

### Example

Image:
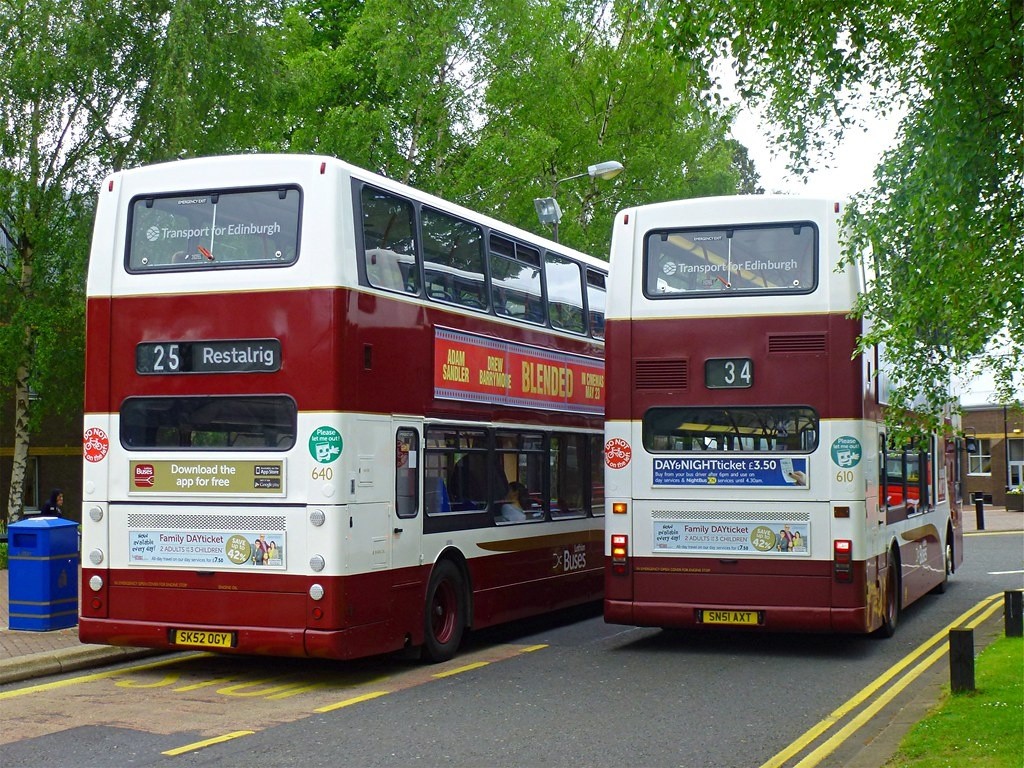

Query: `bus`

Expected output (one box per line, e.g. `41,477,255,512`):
594,191,981,637
75,152,686,663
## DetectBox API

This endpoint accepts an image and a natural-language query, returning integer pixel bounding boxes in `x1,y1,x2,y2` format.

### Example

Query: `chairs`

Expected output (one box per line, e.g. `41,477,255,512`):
405,283,585,334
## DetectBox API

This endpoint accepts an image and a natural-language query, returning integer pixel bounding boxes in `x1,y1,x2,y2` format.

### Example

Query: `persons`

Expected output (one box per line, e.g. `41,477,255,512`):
448,434,530,523
789,471,806,486
41,489,64,518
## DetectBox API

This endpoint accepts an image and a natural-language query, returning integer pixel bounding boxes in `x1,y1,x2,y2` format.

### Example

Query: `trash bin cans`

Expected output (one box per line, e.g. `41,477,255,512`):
6,515,78,632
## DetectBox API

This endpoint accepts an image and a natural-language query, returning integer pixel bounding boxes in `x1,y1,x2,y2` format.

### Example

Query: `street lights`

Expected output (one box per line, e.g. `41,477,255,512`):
533,160,626,244
